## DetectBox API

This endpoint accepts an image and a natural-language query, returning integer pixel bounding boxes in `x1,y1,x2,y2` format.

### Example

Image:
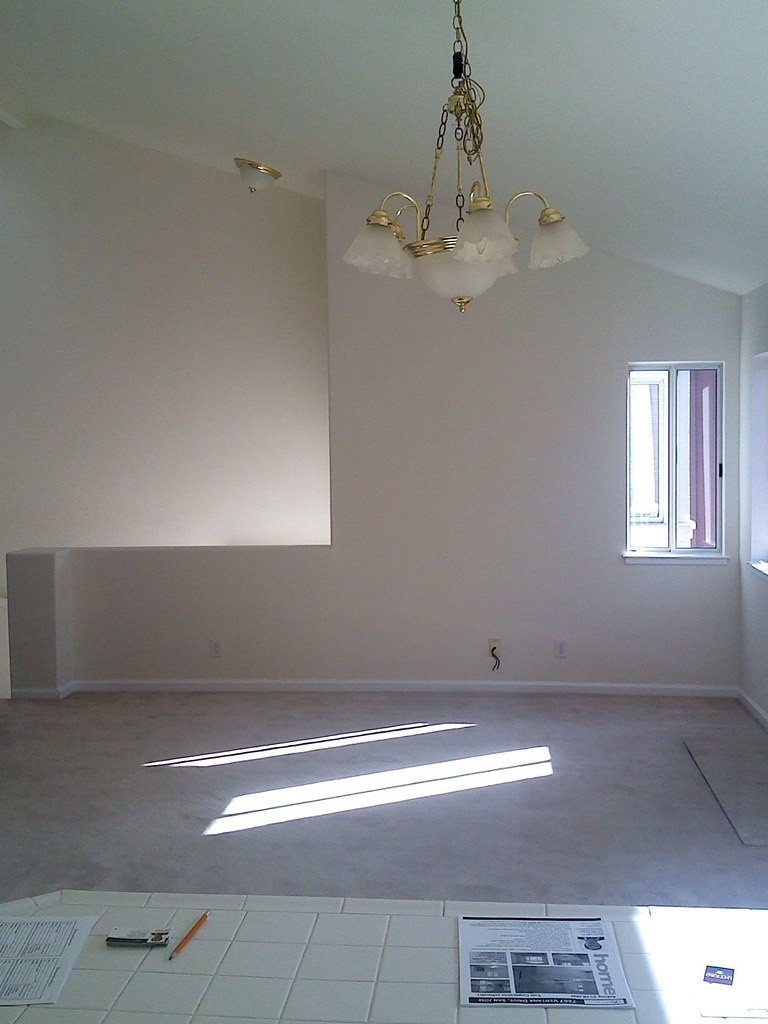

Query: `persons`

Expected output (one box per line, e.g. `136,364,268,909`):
150,932,169,941
577,936,605,951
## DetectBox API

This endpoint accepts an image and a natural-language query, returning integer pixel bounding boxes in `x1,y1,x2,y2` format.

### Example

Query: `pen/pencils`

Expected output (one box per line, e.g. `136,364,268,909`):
167,910,212,961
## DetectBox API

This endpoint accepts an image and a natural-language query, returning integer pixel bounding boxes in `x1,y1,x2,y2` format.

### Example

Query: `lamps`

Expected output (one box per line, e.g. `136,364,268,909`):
232,156,283,195
336,0,592,314
